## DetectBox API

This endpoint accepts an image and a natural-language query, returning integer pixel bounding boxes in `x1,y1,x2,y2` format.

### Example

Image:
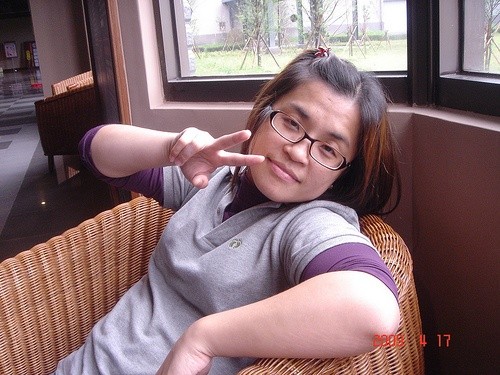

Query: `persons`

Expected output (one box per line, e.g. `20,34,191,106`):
46,47,401,374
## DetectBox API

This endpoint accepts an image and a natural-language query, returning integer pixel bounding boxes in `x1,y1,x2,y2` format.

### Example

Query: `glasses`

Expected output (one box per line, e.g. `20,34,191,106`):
269,101,351,171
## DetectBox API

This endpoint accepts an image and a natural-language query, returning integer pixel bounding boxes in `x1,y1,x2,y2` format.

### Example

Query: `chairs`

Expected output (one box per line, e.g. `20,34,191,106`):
34,84,97,173
0,196,424,375
52,69,93,97
